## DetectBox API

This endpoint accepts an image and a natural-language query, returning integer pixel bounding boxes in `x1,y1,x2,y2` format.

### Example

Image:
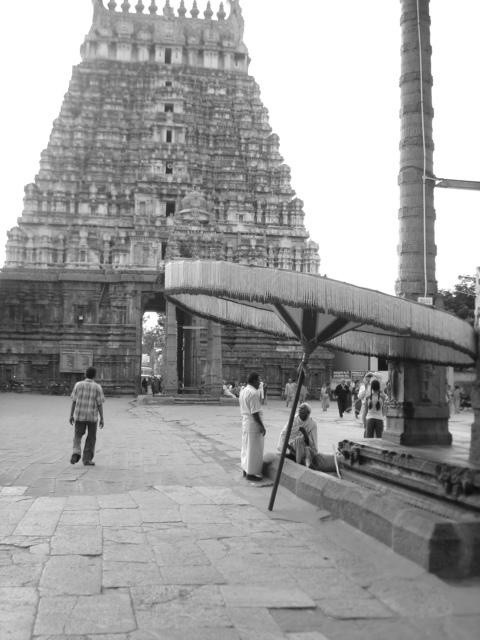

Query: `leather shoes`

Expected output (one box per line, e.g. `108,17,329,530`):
247,475,263,481
70,457,79,465
84,460,95,466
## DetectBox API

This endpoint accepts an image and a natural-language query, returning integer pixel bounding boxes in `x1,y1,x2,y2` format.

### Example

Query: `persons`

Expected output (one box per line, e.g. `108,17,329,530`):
66,366,107,466
445,383,463,417
319,370,389,440
222,378,266,405
238,372,266,482
277,402,319,470
141,376,163,394
284,377,297,406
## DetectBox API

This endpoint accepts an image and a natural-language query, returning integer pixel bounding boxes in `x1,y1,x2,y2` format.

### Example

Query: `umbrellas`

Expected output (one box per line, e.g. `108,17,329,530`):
156,256,478,514
